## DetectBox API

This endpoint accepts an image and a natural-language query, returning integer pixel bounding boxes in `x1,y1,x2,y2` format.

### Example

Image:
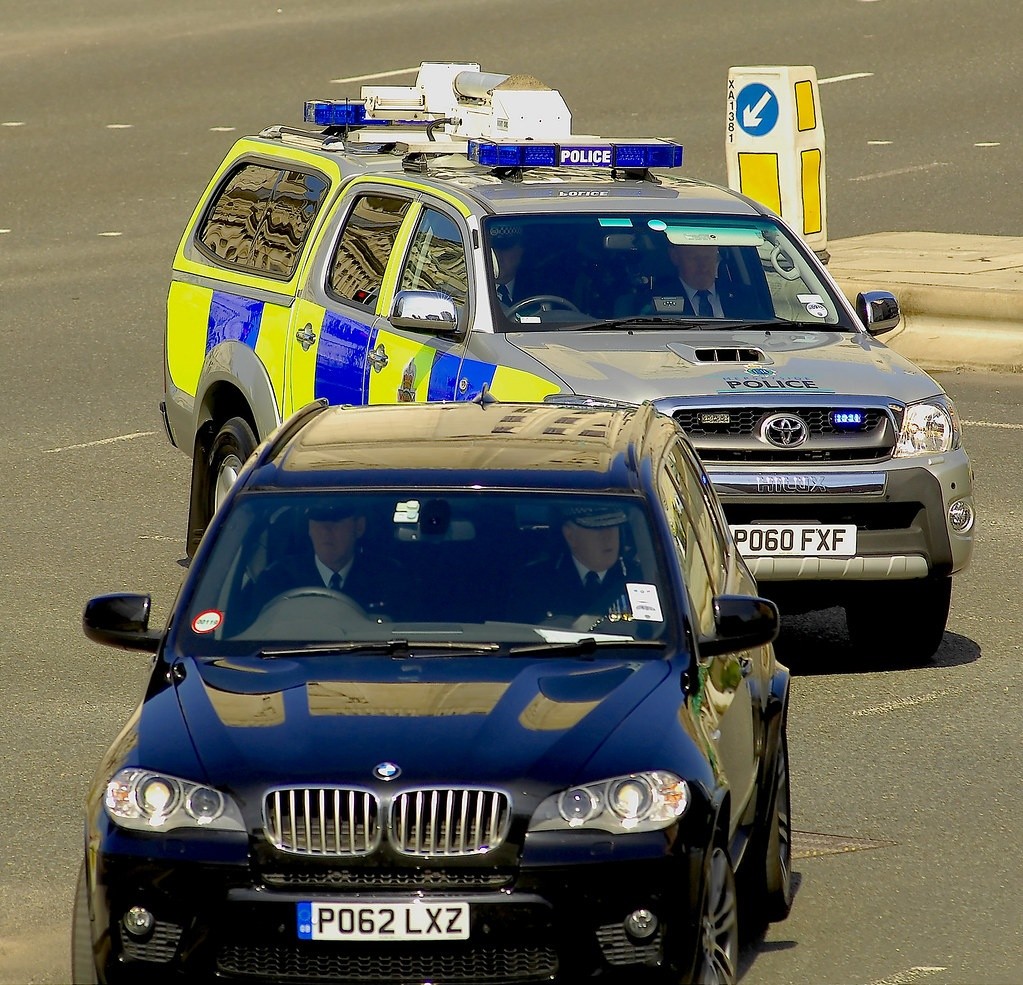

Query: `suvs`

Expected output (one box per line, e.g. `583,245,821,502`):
70,392,791,985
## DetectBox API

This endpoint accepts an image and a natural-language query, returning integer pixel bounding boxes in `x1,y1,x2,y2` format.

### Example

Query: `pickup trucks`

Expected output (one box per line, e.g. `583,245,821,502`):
159,61,976,667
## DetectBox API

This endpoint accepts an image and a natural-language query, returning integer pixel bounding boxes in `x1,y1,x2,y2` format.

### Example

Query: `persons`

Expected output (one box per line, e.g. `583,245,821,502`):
486,218,568,318
643,240,756,318
515,496,653,635
229,489,406,631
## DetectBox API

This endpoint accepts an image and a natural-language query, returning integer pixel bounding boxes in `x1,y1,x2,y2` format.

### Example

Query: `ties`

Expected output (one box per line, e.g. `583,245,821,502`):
584,571,600,591
331,573,342,591
695,289,714,318
496,285,512,308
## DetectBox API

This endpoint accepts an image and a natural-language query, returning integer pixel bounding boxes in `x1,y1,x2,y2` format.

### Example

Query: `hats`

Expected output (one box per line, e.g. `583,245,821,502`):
308,502,354,522
552,500,627,528
489,225,523,249
